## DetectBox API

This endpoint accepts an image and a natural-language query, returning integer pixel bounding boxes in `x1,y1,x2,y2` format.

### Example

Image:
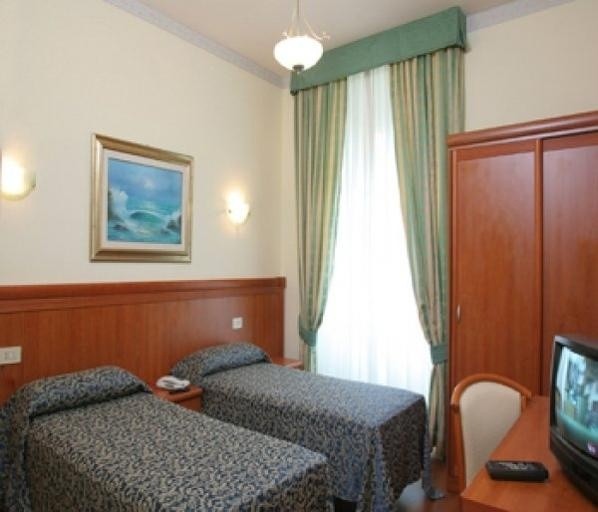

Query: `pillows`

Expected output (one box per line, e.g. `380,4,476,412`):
183,341,267,374
19,365,141,413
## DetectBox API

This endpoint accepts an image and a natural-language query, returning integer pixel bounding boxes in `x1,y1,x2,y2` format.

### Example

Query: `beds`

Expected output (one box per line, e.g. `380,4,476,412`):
178,361,446,512
16,389,334,512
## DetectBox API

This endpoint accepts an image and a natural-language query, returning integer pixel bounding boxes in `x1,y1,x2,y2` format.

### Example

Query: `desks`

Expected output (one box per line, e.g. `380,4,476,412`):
449,373,532,490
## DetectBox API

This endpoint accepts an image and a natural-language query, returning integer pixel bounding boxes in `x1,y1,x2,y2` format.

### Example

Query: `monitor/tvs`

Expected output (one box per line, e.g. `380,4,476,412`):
548,333,598,504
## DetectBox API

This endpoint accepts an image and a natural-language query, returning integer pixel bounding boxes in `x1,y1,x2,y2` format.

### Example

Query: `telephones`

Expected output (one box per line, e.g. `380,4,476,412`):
156,376,190,391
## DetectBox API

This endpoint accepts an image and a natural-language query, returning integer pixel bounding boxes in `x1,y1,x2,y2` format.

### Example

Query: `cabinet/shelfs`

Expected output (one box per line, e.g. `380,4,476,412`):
445,109,598,492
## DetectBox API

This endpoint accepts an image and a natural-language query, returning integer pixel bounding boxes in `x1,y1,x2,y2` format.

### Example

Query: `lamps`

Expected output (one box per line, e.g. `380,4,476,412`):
273,0,327,75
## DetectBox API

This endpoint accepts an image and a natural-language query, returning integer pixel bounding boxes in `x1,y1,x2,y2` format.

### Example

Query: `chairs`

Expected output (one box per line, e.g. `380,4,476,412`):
459,395,597,512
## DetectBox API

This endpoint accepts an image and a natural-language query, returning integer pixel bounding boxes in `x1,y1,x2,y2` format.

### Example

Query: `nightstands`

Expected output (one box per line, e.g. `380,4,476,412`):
151,383,204,411
270,357,303,369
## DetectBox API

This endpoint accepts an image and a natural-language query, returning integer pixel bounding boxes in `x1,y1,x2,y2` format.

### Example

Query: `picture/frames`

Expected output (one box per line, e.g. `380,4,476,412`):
91,132,195,263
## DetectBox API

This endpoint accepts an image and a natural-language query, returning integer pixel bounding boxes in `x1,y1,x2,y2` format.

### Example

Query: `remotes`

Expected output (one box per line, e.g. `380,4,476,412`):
485,460,549,481
